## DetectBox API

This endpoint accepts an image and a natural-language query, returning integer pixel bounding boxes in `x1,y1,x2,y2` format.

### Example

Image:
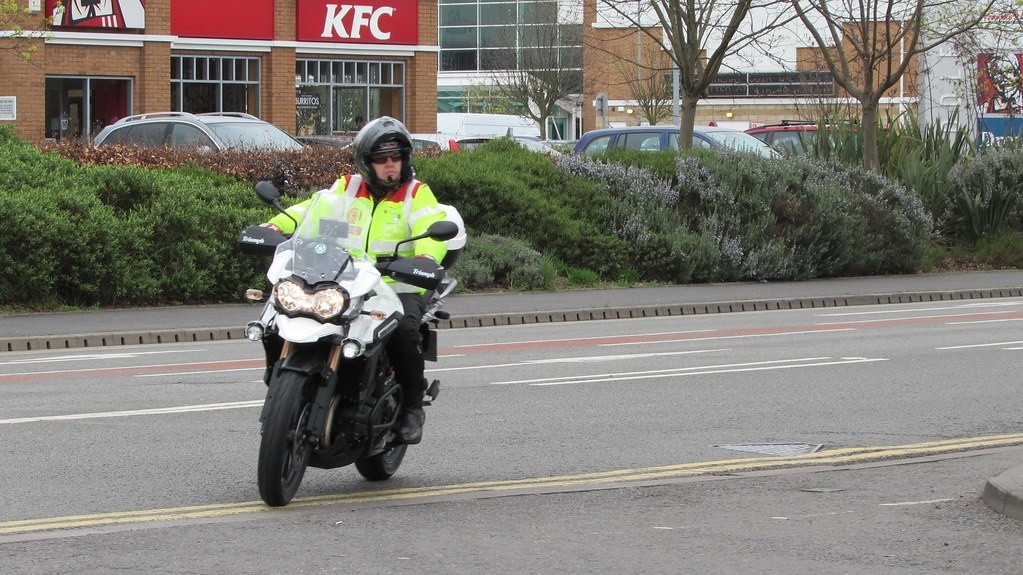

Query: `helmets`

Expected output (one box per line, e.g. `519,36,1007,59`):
352,115,414,186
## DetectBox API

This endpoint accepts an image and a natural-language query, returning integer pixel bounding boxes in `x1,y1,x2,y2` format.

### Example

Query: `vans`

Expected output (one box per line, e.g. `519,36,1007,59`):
436,113,542,138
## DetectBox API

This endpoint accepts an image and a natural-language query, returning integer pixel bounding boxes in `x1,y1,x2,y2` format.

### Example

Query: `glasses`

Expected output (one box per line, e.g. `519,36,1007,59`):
371,152,404,164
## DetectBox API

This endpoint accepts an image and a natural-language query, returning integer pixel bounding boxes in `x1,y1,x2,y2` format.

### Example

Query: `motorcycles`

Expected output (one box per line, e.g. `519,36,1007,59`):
238,181,457,507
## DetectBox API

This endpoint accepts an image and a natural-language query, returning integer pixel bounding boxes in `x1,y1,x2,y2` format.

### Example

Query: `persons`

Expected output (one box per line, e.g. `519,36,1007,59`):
256,115,449,442
354,116,365,131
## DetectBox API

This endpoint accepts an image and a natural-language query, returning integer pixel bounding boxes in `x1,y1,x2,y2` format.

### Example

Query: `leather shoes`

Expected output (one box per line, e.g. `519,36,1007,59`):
400,405,423,444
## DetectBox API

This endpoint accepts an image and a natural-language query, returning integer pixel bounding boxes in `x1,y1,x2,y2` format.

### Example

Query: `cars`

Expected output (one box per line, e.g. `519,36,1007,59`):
330,132,561,156
574,127,783,159
294,136,354,152
542,140,579,148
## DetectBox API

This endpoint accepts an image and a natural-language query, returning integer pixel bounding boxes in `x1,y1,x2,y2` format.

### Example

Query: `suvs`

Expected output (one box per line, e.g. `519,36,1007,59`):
89,112,312,183
742,119,889,157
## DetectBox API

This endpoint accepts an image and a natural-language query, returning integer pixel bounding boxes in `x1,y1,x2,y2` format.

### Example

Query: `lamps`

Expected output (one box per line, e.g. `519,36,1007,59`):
726,113,733,117
626,108,634,114
